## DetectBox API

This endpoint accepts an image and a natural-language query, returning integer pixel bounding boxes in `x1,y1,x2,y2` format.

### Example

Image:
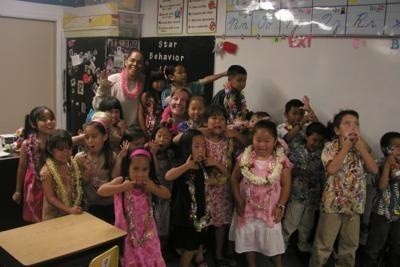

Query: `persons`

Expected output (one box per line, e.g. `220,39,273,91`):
13,51,400,266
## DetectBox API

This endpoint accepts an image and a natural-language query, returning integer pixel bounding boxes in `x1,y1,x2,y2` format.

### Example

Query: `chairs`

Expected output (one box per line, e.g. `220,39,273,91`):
88,244,120,266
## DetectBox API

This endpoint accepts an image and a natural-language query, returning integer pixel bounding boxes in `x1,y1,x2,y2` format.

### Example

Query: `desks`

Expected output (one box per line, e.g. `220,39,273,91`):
0,157,20,164
0,210,128,267
